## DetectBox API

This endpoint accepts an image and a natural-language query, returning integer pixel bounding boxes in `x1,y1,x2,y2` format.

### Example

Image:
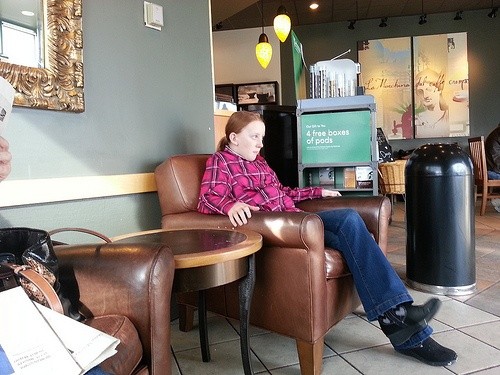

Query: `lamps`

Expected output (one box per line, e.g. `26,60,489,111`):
488,7,499,19
255,0,272,69
418,14,427,25
273,0,291,43
379,16,388,28
347,19,356,30
454,10,464,20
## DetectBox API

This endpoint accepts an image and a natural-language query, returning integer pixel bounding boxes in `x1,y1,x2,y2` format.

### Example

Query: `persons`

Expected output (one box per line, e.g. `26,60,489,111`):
195,111,458,367
484,125,500,213
0,136,12,182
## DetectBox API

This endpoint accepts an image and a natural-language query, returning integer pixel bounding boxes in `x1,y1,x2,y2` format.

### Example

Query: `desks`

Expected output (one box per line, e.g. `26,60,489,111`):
103,228,263,375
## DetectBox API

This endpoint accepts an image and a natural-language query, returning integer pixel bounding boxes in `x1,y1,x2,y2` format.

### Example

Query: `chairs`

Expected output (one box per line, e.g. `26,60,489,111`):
468,135,500,217
155,154,391,375
54,242,175,375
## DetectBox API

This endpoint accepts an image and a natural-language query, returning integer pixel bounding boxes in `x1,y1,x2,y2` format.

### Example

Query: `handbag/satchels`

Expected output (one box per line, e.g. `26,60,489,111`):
0,222,111,323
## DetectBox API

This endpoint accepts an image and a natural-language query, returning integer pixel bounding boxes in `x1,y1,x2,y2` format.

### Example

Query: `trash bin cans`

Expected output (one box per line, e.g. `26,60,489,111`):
403,140,478,297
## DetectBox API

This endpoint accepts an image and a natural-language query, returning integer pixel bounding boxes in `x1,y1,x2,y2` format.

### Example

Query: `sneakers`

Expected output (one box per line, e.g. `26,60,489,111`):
378,298,440,342
393,335,457,366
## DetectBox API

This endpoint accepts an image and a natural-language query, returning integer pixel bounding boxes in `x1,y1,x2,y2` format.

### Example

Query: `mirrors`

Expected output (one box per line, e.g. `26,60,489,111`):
0,0,85,114
